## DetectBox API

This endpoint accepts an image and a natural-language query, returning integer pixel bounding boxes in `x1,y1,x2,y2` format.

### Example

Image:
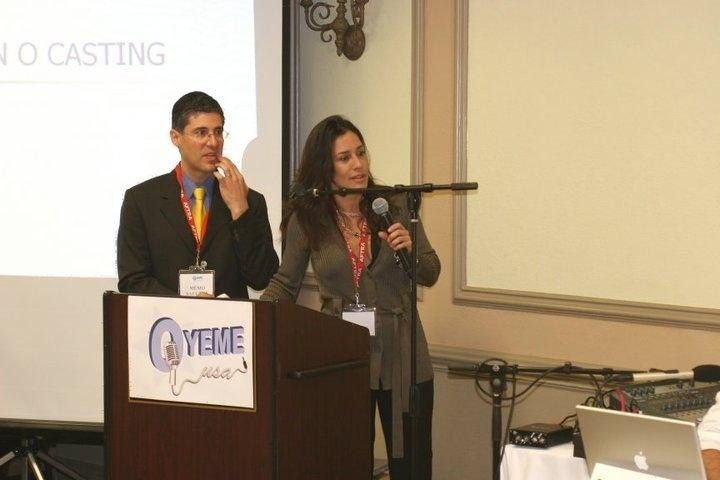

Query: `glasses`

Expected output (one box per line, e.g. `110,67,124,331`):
176,127,232,142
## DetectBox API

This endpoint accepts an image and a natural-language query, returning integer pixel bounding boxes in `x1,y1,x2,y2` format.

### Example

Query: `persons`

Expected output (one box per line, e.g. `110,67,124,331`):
115,89,279,299
696,392,720,480
258,113,441,479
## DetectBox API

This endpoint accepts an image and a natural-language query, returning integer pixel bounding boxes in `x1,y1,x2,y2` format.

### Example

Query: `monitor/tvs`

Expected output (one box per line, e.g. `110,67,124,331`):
574,404,707,480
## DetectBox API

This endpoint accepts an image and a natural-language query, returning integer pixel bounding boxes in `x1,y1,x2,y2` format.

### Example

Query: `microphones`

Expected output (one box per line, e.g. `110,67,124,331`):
612,364,720,384
371,197,412,274
303,187,321,202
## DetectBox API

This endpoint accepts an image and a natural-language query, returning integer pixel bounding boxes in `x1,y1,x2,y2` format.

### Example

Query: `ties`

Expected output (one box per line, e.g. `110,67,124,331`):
191,187,211,243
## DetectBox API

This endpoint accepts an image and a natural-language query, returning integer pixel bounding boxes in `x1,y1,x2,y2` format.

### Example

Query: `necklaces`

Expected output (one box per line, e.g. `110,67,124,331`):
338,210,362,218
340,222,361,238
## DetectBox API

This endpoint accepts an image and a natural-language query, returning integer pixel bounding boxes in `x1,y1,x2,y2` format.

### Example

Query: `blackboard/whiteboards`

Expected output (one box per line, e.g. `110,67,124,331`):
291,1,423,290
452,1,720,333
